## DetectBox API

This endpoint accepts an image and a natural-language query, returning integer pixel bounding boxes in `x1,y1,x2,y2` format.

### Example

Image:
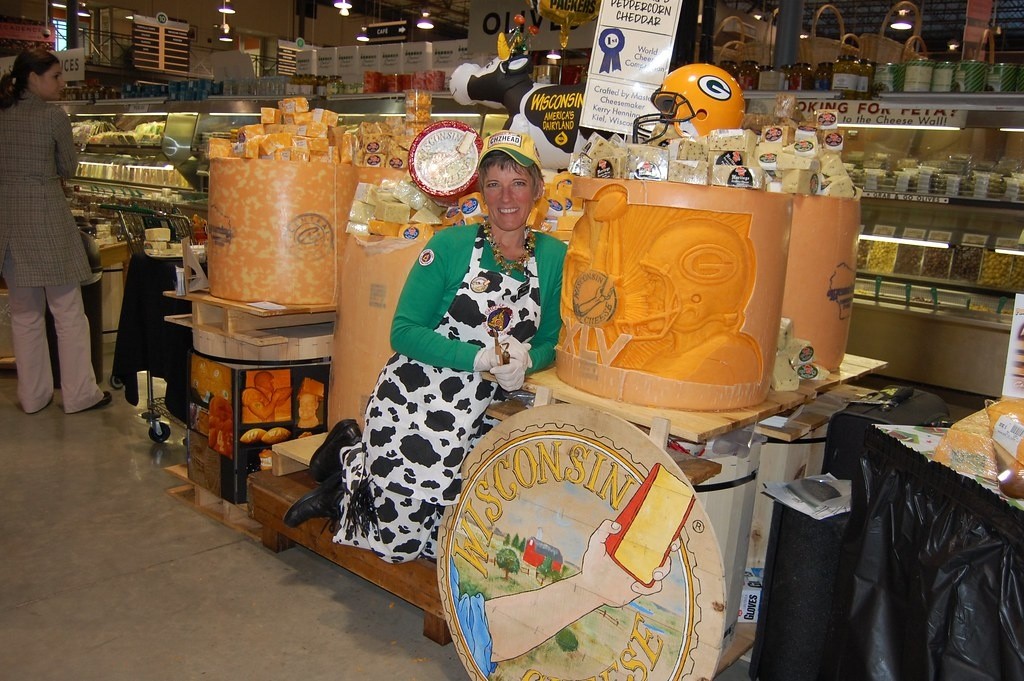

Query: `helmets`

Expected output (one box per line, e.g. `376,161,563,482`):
632,64,744,145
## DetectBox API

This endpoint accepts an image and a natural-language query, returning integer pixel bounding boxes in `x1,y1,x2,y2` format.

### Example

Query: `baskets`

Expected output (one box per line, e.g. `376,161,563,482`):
737,8,779,68
712,16,744,62
794,5,861,64
859,2,927,63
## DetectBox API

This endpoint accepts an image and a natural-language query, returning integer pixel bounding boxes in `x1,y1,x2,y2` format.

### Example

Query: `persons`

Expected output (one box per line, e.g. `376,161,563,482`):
282,128,570,562
0,50,112,414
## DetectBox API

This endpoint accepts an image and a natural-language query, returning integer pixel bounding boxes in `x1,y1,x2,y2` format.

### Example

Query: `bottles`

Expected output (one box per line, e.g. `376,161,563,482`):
228,72,287,95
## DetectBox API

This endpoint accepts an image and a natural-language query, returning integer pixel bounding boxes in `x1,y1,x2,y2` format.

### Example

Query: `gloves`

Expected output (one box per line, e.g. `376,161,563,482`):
473,336,533,372
490,358,527,392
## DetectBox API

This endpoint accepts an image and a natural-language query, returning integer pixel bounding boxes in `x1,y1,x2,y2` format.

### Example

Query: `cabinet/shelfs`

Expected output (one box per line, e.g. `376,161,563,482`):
745,95,1023,398
53,100,330,233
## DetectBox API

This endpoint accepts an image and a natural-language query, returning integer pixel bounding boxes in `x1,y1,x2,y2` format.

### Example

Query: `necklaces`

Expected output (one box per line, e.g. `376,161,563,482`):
482,222,535,274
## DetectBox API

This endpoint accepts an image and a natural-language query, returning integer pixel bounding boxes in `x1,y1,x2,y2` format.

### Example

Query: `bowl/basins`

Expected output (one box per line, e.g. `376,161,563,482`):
402,89,433,138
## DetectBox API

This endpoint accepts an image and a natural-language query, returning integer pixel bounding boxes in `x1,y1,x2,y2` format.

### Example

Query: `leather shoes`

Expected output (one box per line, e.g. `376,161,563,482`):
309,419,363,484
282,469,352,536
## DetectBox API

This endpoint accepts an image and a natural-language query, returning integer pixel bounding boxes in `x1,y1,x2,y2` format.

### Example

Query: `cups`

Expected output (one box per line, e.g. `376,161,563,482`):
363,69,446,95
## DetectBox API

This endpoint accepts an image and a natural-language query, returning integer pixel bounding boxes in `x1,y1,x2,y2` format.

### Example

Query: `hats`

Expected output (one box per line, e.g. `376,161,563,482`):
476,130,541,173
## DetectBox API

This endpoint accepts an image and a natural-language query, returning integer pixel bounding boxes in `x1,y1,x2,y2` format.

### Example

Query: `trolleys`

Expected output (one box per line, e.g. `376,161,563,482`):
96,200,207,448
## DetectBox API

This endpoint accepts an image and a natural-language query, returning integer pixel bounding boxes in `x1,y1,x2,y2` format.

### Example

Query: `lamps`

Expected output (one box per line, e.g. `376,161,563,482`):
77,0,90,17
356,0,369,41
414,0,434,29
217,0,236,42
892,7,914,30
333,0,352,16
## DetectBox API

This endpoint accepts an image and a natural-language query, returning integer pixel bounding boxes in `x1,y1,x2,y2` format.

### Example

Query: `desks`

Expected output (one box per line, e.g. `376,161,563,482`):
110,253,206,445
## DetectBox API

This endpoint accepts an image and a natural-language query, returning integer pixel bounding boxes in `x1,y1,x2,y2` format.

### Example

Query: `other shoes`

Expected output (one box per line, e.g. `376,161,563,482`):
68,391,112,415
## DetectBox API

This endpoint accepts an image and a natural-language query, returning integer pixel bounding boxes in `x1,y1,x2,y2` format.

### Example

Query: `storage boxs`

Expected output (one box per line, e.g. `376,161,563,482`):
296,39,468,83
